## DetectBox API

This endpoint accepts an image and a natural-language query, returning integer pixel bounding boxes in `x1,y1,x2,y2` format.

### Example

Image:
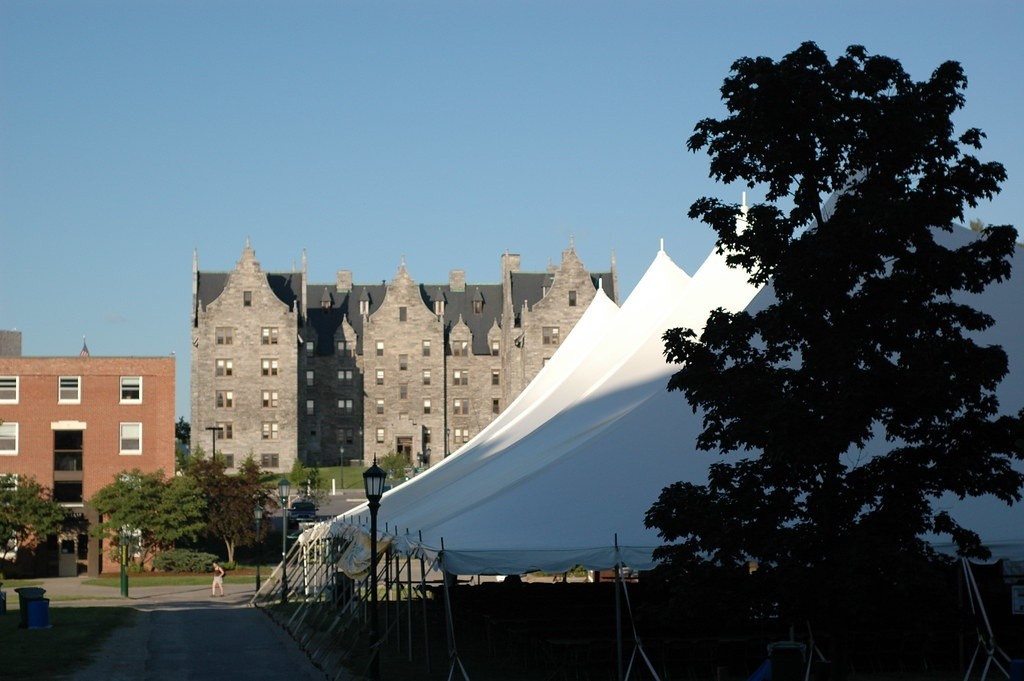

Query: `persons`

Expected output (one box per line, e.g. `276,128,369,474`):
209,561,225,597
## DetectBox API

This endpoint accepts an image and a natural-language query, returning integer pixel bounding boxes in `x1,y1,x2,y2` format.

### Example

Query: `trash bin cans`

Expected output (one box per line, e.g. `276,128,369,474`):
14,583,53,629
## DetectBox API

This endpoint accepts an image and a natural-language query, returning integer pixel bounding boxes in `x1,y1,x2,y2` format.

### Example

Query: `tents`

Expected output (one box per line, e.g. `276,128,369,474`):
249,159,1024,681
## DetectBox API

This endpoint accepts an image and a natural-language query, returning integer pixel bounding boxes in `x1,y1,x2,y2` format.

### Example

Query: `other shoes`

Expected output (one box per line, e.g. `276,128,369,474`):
209,595,216,598
219,595,224,597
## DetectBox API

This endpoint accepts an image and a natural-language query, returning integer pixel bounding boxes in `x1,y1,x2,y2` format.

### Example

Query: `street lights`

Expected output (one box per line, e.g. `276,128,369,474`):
363,454,387,681
278,479,293,603
253,503,264,592
340,446,344,489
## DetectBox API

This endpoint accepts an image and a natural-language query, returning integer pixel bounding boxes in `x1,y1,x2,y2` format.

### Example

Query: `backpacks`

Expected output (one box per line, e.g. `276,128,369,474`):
218,566,226,577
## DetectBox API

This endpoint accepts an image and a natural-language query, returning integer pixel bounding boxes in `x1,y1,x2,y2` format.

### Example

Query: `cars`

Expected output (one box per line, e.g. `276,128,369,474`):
286,515,338,553
288,501,319,527
